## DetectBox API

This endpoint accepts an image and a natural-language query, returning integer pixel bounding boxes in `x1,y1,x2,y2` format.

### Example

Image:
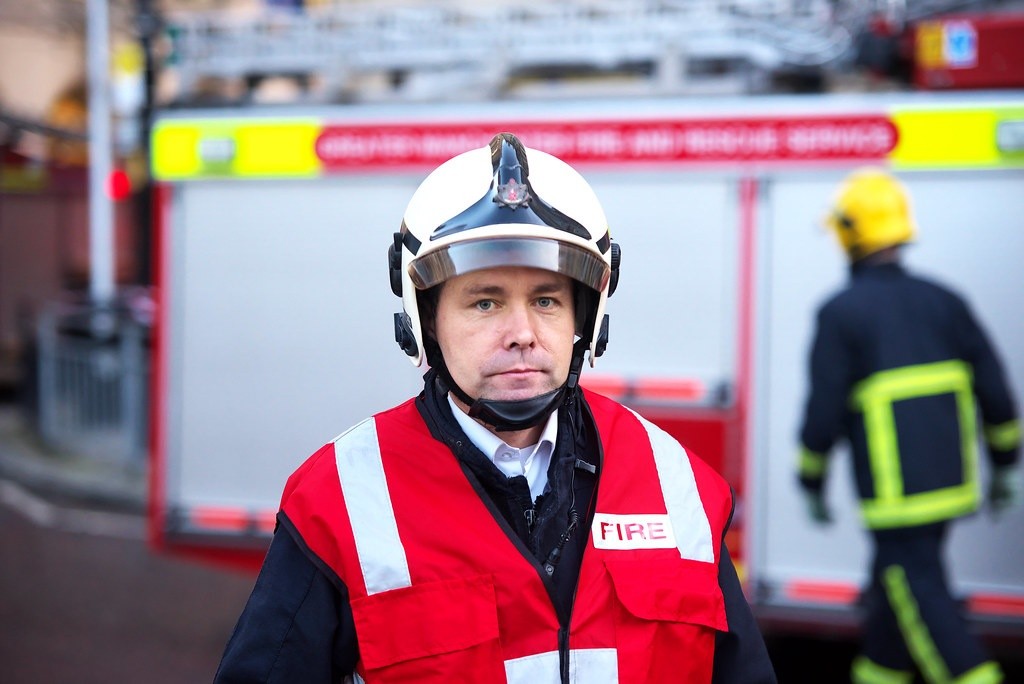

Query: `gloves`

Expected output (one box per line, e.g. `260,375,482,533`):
989,467,1017,509
799,474,832,523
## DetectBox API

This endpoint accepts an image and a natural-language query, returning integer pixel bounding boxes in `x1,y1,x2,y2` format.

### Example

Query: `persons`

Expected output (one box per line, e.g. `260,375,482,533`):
217,132,778,684
794,164,1024,683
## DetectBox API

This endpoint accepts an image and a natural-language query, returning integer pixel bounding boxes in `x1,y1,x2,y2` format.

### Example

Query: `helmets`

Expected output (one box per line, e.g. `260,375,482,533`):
831,173,913,260
388,132,621,368
